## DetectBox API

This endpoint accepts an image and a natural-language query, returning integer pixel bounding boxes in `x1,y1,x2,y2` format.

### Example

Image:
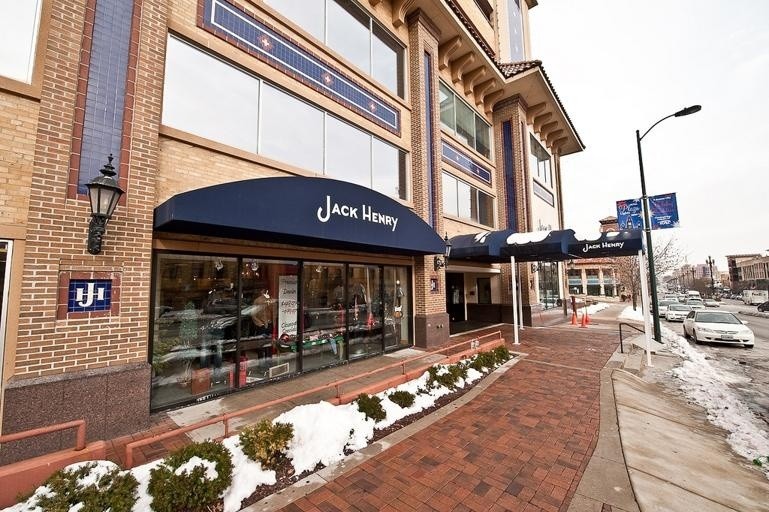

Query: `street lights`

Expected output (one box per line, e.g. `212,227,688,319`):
636,105,702,342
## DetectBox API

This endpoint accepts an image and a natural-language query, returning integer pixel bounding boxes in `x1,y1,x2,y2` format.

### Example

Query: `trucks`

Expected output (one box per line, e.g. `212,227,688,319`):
743,289,769,305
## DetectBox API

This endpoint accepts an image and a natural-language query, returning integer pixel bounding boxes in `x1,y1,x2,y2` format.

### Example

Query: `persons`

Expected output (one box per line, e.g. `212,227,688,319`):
252,288,272,372
199,292,225,384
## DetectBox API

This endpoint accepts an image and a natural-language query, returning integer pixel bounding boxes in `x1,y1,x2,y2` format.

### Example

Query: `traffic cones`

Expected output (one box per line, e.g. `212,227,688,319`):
572,312,577,324
581,313,590,328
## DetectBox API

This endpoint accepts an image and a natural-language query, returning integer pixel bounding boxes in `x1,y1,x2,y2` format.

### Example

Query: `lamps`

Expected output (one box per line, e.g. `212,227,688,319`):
434,229,453,271
82,150,127,258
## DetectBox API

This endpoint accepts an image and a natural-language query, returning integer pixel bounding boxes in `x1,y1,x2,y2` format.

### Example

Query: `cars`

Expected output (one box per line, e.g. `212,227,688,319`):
208,298,314,340
756,301,768,312
682,311,754,346
649,289,719,321
